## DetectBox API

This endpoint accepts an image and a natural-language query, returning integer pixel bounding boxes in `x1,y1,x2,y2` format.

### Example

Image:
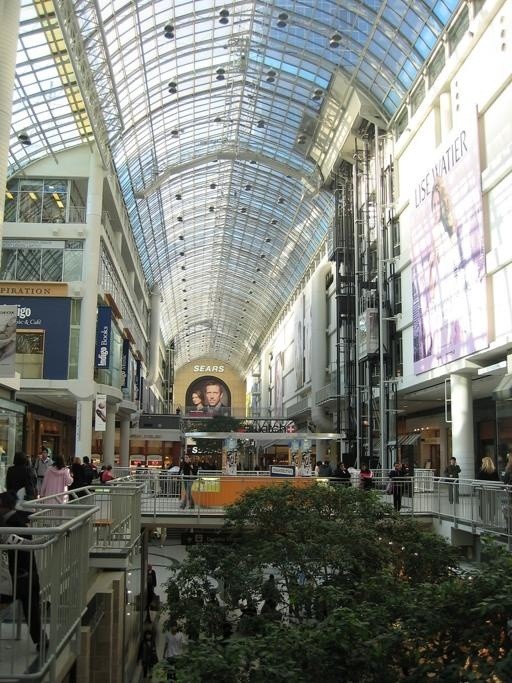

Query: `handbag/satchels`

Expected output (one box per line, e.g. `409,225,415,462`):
15,487,40,513
386,478,394,495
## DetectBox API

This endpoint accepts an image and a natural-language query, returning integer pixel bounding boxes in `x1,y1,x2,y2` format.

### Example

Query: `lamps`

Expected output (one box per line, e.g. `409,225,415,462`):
18,129,31,147
164,6,342,145
175,183,284,294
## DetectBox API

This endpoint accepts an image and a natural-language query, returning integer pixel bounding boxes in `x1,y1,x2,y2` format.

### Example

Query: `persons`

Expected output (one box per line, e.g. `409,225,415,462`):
136,453,217,509
478,457,499,520
314,461,374,492
389,462,407,512
238,462,260,470
429,182,468,353
191,390,205,411
168,565,312,638
443,457,461,505
147,565,157,593
136,622,186,681
203,382,227,413
1,447,113,653
